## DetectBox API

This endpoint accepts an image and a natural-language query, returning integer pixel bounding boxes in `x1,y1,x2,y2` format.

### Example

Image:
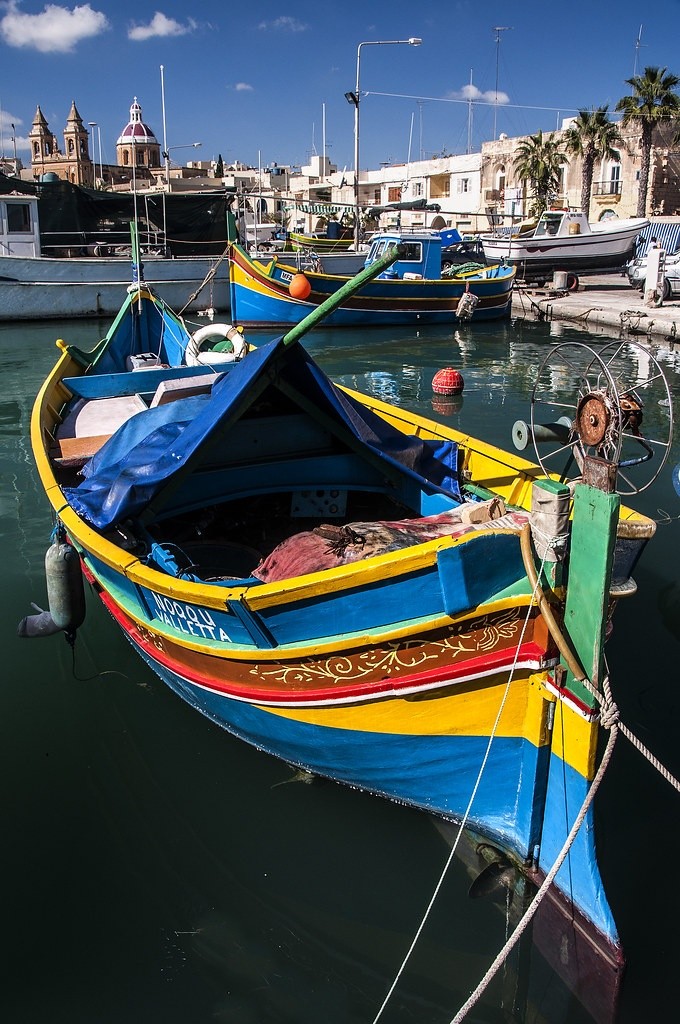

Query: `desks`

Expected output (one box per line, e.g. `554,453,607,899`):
49,432,112,467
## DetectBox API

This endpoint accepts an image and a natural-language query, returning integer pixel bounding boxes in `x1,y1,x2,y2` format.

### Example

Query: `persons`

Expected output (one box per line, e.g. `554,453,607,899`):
619,232,641,277
646,236,657,256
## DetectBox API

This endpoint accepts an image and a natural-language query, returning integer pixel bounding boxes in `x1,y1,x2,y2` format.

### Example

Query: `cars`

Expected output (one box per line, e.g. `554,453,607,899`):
625,247,680,299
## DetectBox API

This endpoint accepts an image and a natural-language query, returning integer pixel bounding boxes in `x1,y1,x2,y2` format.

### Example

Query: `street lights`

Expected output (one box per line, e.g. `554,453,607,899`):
11,123,18,158
343,38,426,254
494,26,513,142
87,121,99,189
160,141,202,183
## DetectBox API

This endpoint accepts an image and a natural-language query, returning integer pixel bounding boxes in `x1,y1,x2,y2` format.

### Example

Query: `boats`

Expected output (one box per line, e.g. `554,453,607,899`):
481,208,651,293
0,171,238,323
219,100,516,328
28,245,655,1024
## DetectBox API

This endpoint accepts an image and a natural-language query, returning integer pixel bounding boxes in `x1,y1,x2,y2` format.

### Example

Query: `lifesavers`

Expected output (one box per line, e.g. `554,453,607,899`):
185,321,247,367
